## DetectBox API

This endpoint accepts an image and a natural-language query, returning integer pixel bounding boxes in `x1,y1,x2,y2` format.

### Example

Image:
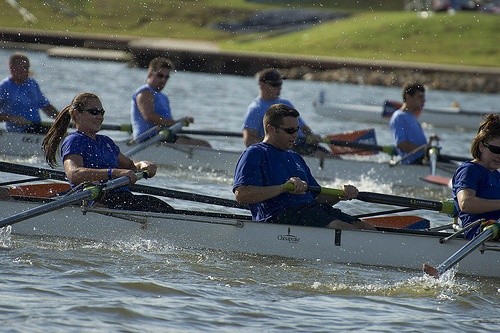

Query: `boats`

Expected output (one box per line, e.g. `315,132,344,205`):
311,90,493,133
1,119,471,197
0,162,500,278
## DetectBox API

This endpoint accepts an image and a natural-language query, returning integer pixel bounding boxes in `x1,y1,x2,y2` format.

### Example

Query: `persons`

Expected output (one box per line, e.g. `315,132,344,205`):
242,69,341,159
390,82,430,163
451,114,500,243
42,92,178,214
0,53,60,134
130,58,212,147
234,105,376,231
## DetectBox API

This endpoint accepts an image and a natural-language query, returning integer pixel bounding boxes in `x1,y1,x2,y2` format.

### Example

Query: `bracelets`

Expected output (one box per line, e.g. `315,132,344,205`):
108,168,112,178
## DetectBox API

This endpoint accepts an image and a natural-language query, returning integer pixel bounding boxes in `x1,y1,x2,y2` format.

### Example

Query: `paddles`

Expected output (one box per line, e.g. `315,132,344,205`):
0,159,253,212
297,133,397,156
279,178,461,217
381,99,495,118
0,162,157,229
421,217,500,281
1,116,380,159
424,133,453,186
0,160,430,241
122,116,194,158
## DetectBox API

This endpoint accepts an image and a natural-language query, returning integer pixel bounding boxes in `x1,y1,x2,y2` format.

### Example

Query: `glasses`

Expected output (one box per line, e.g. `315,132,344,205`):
275,125,300,134
82,108,105,116
483,142,500,154
154,71,170,79
265,81,282,87
